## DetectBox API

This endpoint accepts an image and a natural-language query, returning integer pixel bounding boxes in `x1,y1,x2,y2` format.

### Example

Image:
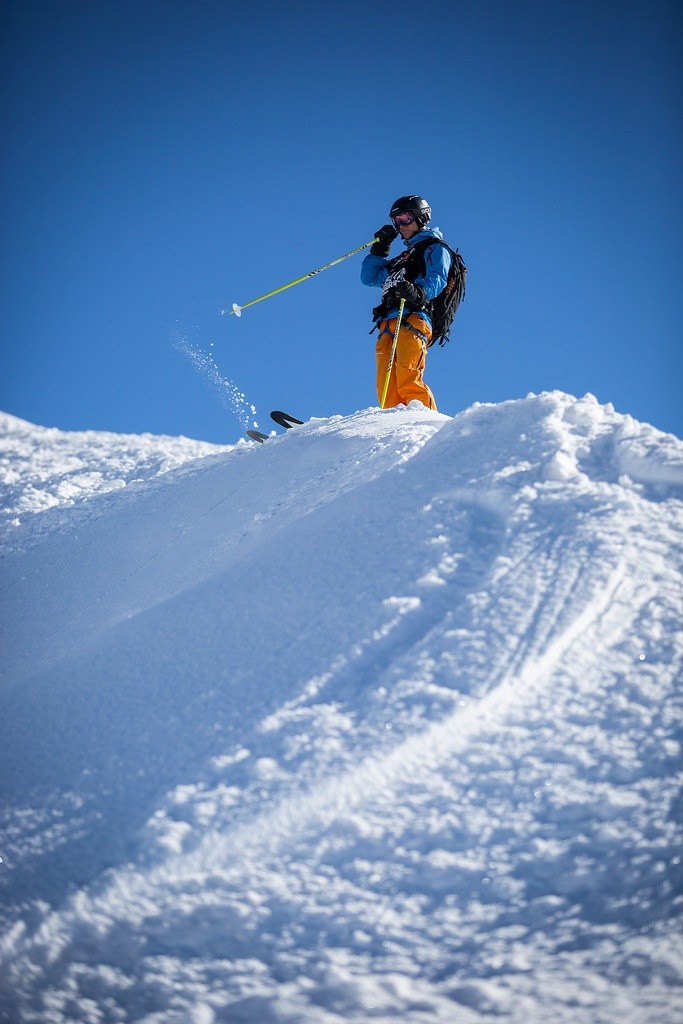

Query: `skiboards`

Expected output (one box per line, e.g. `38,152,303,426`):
247,408,306,443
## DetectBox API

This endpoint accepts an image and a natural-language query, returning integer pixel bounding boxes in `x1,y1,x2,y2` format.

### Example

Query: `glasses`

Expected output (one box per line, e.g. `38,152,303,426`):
391,211,415,230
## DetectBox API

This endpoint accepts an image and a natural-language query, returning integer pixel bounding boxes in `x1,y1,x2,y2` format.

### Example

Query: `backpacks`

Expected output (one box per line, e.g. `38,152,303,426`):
389,243,468,347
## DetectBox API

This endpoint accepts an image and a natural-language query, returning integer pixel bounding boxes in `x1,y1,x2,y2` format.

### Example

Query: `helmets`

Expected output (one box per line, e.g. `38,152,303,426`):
389,195,431,228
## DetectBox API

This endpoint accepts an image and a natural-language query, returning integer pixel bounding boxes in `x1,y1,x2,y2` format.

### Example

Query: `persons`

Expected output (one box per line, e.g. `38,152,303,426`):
360,193,451,412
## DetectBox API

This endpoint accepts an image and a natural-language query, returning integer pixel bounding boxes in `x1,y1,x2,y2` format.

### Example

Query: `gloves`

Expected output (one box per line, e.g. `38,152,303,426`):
400,280,426,306
370,225,398,256
